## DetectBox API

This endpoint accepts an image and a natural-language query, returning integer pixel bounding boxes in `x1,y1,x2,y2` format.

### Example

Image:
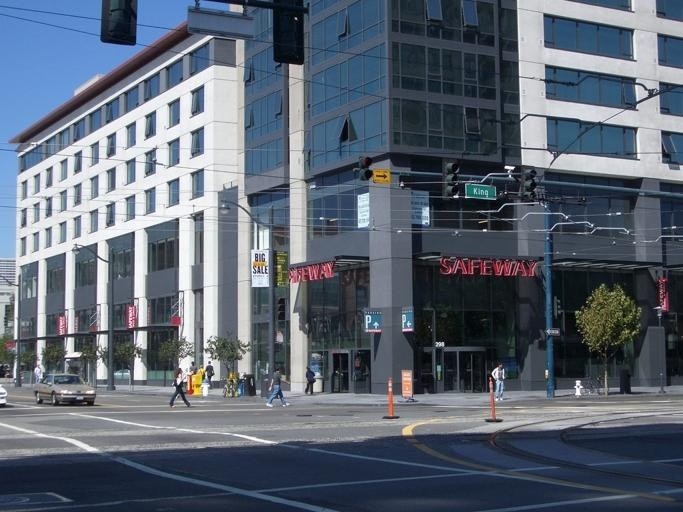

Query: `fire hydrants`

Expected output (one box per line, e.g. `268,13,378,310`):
200,379,210,397
573,379,583,396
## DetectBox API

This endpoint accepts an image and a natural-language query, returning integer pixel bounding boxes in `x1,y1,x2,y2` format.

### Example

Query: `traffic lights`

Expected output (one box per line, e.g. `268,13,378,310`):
520,165,536,205
442,158,460,201
358,156,372,182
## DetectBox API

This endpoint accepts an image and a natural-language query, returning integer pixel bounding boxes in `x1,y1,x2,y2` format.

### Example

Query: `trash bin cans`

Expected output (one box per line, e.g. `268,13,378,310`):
243,374,256,396
260,373,268,398
619,368,631,393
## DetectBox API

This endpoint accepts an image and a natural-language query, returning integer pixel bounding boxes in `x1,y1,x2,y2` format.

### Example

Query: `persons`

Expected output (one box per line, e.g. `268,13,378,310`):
305,366,315,394
34,364,46,384
205,361,213,389
189,361,197,374
169,368,190,408
491,362,506,401
265,367,291,408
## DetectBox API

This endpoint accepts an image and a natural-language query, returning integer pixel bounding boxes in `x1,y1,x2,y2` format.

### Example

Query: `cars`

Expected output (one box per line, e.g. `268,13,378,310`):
33,373,95,406
0,384,7,405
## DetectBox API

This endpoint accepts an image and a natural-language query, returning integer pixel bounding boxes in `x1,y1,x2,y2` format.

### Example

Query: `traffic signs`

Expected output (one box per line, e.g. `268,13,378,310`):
545,327,560,338
373,170,391,184
464,184,497,201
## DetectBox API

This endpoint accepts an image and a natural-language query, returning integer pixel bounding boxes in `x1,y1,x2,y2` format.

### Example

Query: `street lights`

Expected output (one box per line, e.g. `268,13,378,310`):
218,197,276,397
71,242,114,391
652,305,666,394
